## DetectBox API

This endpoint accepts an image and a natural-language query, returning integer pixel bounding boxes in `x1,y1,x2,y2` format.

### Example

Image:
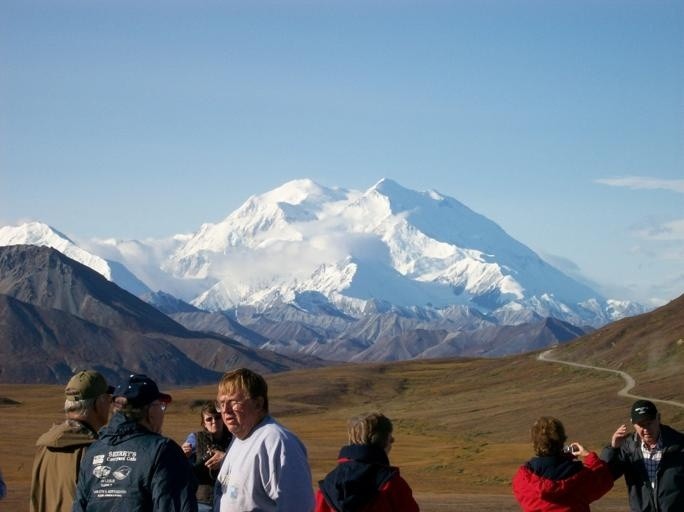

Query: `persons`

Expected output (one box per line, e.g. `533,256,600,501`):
212,368,315,511
313,412,420,511
70,374,199,512
600,399,683,511
511,415,615,511
27,369,115,511
180,400,232,511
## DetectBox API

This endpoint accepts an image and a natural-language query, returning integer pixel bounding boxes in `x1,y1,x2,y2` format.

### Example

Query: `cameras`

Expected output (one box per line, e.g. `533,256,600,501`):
563,444,579,460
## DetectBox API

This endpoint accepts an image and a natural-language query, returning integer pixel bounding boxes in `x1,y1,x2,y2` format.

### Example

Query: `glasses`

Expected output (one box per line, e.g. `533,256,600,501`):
214,399,241,413
206,415,221,422
389,437,394,444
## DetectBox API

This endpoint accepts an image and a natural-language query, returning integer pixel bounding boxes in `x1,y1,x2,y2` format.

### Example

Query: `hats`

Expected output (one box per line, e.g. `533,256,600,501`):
64,370,172,405
631,400,657,424
